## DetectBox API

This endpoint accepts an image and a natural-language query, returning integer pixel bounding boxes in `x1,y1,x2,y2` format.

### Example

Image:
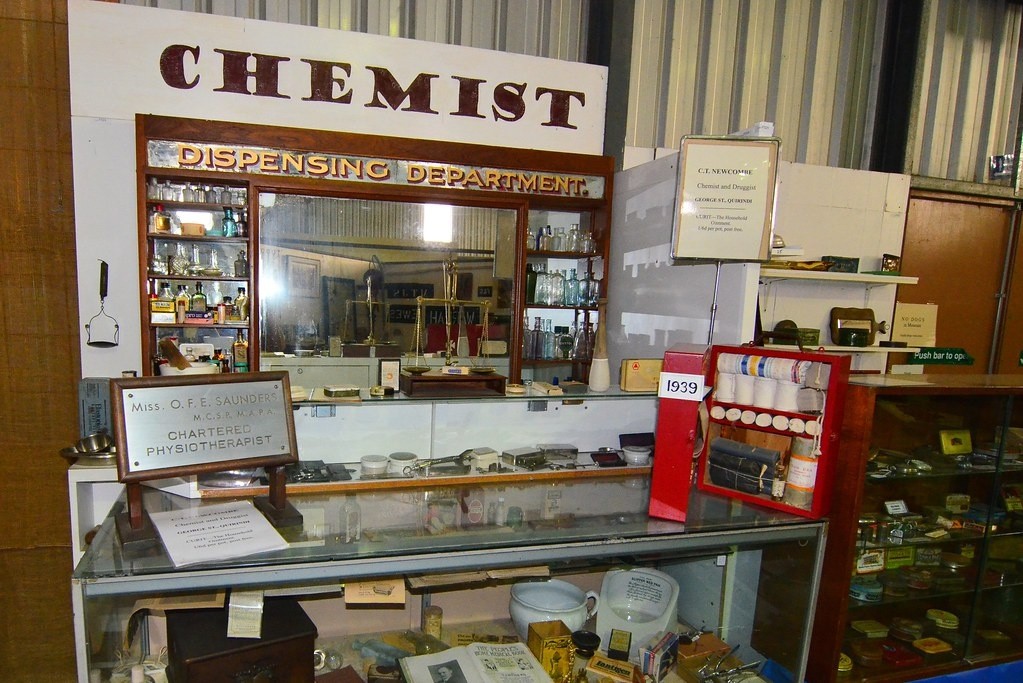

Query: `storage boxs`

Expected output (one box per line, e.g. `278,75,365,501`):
165,597,318,683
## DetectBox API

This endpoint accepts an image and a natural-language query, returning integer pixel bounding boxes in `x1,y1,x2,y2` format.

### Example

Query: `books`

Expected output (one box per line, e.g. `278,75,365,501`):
398,642,554,683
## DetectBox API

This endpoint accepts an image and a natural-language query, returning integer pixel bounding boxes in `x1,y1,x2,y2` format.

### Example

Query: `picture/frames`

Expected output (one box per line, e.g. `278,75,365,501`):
282,254,322,300
319,275,359,346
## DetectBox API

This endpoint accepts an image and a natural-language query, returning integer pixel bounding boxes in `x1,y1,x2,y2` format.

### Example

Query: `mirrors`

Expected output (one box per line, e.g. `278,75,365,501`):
257,191,515,386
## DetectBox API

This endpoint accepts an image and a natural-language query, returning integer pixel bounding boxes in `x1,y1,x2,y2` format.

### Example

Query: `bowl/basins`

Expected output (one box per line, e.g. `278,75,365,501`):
571,630,602,659
509,578,598,640
75,433,112,453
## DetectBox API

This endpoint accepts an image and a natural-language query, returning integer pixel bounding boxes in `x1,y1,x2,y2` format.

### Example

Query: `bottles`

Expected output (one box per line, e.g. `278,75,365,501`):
422,605,443,640
487,503,495,525
404,630,451,655
147,177,248,237
495,498,506,526
461,484,485,529
521,316,595,359
148,281,249,325
184,328,249,374
339,493,361,544
507,506,522,527
352,638,413,666
526,224,597,253
150,239,249,277
525,263,600,308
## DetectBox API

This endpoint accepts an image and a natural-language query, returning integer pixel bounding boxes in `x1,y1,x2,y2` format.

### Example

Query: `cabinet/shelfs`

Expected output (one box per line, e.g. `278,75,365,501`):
616,121,1023,682
519,195,611,382
134,164,251,380
67,459,830,682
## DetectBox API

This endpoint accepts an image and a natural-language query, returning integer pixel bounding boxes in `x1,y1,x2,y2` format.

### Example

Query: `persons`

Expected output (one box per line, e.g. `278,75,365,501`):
435,666,465,683
